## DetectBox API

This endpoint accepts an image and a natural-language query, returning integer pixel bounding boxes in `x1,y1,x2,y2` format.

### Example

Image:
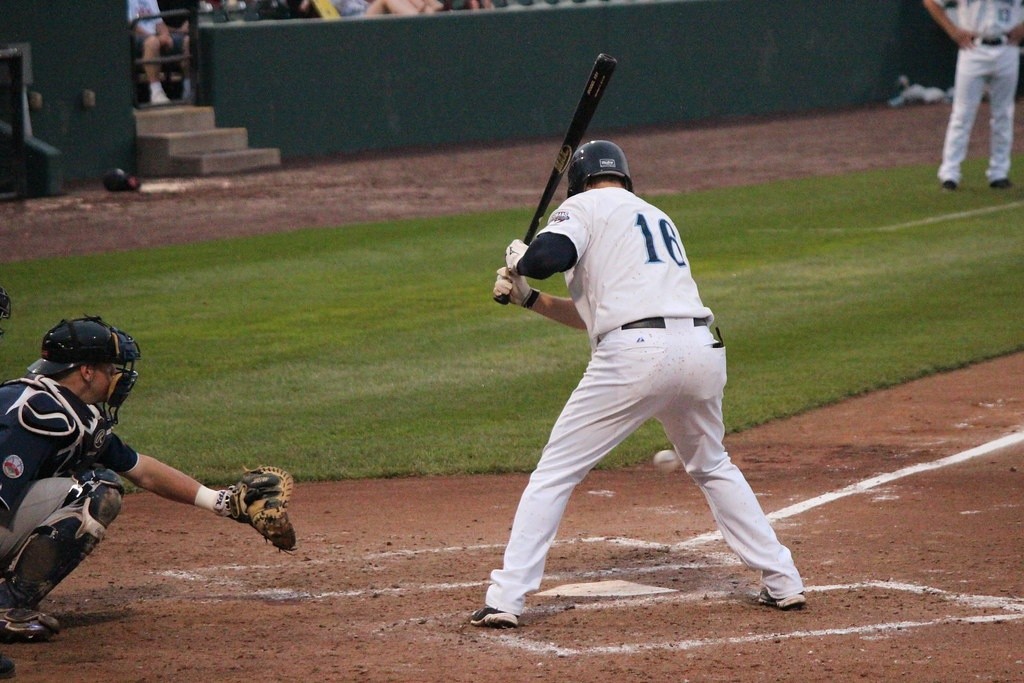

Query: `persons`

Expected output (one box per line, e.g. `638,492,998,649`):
471,139,806,627
0,315,296,644
127,0,494,106
923,1,1023,189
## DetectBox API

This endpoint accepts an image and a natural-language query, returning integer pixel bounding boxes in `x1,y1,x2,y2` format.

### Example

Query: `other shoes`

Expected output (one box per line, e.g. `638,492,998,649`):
0,654,15,677
990,178,1014,189
943,181,957,191
151,86,171,105
0,609,53,642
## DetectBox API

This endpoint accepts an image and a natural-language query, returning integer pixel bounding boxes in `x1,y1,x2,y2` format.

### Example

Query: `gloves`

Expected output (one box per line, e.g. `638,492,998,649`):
493,267,540,308
505,239,528,276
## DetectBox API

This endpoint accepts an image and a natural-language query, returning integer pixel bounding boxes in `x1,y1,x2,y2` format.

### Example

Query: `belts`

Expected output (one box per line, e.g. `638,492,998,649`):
622,317,707,329
982,38,1001,46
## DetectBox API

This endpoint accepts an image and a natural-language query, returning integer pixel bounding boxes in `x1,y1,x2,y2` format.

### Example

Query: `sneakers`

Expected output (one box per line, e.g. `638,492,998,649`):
471,606,518,627
759,586,806,608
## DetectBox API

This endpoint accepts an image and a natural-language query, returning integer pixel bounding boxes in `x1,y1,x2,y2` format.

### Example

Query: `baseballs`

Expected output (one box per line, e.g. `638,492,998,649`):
493,55,619,306
653,449,679,474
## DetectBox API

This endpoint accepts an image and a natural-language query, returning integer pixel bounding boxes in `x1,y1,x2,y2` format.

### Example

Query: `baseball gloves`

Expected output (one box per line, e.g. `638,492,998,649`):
231,466,296,553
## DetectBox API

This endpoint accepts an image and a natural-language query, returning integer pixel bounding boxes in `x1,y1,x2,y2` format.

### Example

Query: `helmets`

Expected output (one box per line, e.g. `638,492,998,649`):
567,140,633,198
28,318,115,375
